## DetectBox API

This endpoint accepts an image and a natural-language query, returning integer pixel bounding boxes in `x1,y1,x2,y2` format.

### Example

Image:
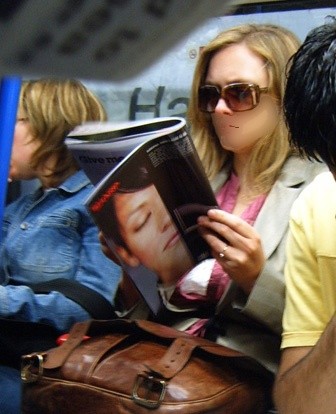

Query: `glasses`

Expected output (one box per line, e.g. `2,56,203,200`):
197,83,270,112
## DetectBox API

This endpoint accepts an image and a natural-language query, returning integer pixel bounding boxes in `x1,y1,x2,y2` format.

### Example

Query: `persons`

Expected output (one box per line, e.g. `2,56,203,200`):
270,14,336,414
0,77,139,414
96,21,332,375
96,159,194,313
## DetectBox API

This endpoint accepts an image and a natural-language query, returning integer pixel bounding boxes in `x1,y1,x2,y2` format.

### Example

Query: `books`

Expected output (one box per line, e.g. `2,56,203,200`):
62,116,227,317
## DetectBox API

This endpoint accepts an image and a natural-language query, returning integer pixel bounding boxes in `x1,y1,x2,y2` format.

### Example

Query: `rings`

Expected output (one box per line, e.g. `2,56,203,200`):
219,246,228,258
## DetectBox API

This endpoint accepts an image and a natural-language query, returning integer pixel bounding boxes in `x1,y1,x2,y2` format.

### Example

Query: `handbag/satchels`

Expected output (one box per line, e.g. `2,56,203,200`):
21,317,277,414
